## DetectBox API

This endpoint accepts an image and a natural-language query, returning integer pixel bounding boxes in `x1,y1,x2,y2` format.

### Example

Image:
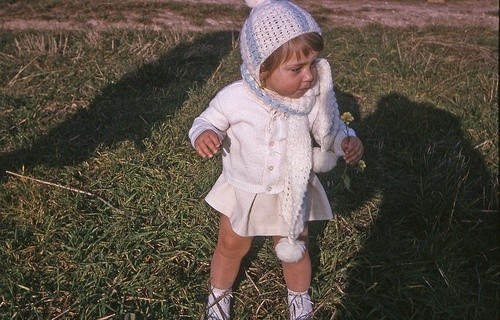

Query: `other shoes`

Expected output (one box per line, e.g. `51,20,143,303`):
207,284,233,320
287,288,313,320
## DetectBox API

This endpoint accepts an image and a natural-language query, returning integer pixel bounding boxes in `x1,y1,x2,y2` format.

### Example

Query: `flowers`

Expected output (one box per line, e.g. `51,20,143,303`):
340,111,367,174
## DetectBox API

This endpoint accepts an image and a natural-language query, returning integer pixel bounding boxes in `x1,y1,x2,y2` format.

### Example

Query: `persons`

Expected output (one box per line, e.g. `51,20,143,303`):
189,1,364,320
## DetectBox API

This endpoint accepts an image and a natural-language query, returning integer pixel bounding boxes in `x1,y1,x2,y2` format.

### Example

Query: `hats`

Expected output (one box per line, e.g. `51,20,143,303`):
239,0,322,89
